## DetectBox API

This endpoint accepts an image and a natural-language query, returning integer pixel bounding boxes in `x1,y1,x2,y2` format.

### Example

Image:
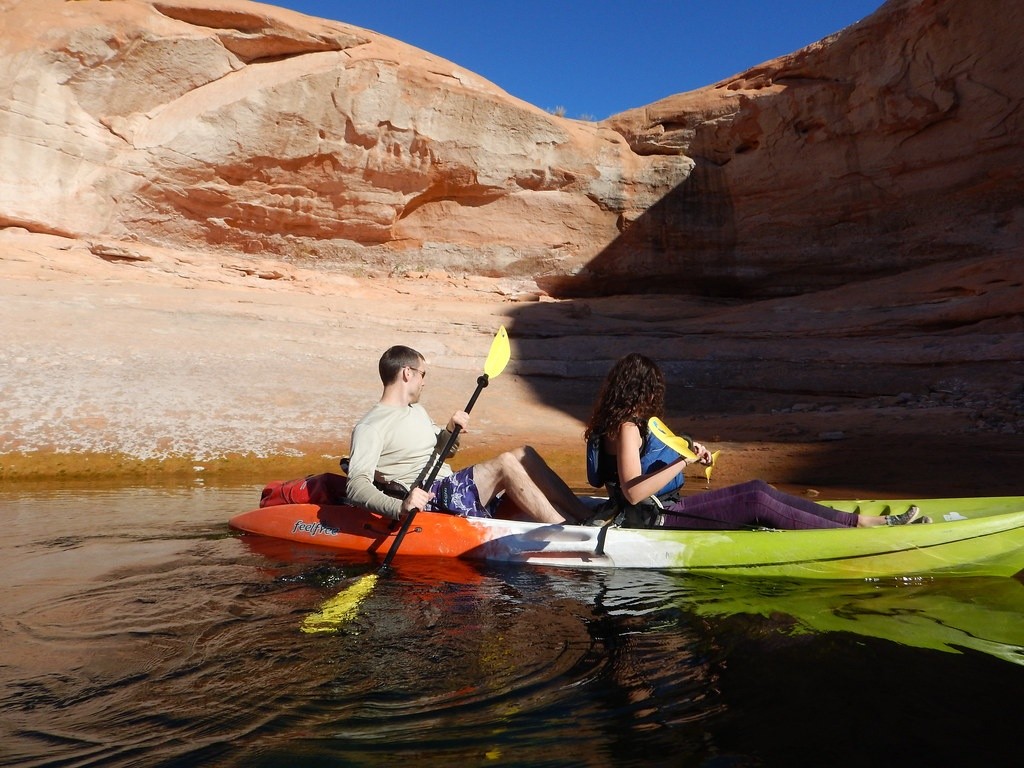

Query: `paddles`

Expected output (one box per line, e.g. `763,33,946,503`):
648,417,721,484
299,325,512,634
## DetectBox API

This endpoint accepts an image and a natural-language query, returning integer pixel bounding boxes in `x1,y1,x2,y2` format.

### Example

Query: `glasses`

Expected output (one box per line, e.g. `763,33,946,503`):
403,366,426,379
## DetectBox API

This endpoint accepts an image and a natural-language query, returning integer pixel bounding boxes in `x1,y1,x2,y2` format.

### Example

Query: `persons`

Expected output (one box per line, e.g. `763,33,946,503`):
345,344,615,527
582,350,934,531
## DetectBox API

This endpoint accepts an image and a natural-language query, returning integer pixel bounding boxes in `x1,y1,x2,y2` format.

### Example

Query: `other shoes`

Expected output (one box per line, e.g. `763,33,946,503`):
583,498,630,529
885,505,932,525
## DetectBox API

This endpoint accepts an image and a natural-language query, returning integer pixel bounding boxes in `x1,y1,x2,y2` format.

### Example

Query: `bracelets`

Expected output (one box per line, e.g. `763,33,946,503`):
685,458,690,466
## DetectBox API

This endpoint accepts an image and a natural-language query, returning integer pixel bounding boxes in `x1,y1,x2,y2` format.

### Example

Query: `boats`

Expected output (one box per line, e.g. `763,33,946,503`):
227,493,1024,580
